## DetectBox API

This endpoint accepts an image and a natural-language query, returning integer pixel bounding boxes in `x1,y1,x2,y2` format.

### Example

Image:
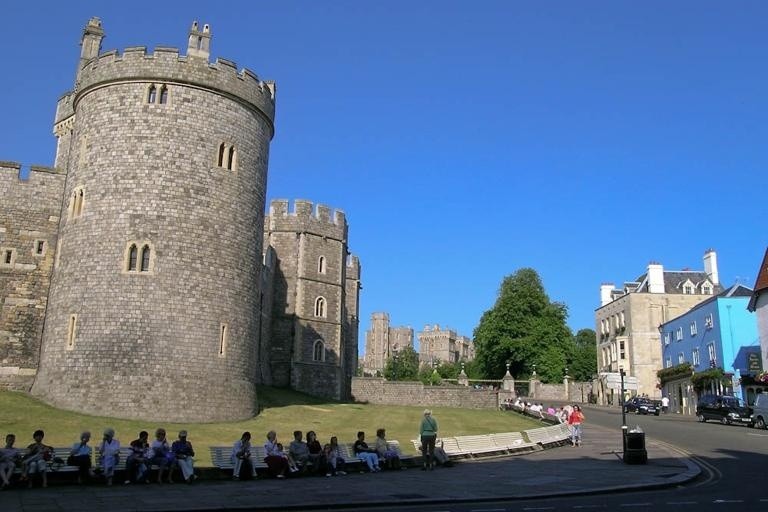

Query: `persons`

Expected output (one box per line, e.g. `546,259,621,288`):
637,393,648,398
420,409,438,471
504,397,574,420
354,432,381,472
265,429,347,478
232,432,257,479
568,405,584,447
1,427,198,486
662,395,669,414
375,429,400,471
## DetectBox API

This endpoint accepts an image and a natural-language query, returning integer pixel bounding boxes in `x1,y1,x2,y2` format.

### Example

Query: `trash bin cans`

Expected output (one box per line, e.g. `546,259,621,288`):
626,433,647,464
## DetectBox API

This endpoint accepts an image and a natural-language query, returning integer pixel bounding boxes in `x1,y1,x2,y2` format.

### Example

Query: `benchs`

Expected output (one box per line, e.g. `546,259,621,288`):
346,440,415,470
285,444,361,471
410,401,578,462
0,447,92,482
94,445,192,481
209,446,286,475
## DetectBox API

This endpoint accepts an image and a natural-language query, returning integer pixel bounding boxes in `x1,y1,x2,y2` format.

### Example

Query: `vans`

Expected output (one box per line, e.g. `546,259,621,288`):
750,391,768,428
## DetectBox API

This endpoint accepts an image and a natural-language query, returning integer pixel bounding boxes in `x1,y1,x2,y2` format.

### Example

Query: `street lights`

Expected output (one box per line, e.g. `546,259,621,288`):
618,362,627,453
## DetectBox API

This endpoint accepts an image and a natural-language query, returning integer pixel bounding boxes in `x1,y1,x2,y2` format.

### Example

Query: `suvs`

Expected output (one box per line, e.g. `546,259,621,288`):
696,395,756,428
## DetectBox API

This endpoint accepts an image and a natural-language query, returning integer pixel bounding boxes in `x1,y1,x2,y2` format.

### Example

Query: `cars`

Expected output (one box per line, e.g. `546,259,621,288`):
624,396,660,416
494,385,520,398
468,382,486,390
